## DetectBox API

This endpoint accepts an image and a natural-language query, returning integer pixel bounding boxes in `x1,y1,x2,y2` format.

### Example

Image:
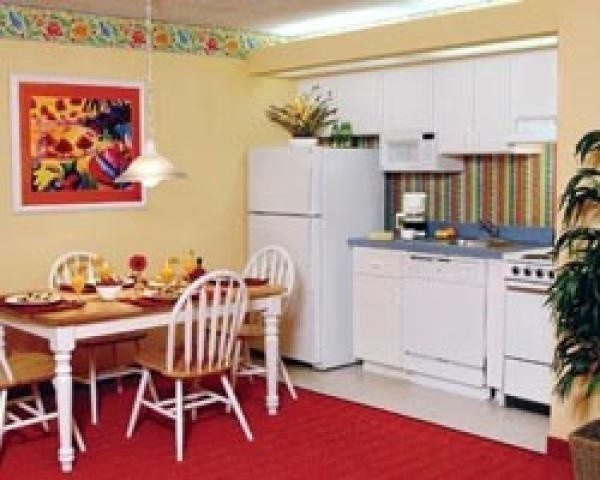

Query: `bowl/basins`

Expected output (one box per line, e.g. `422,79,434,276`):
94,286,122,302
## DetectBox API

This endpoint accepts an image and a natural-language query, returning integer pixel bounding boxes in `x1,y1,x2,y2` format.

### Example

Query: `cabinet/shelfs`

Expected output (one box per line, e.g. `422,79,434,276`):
296,47,558,157
349,247,491,402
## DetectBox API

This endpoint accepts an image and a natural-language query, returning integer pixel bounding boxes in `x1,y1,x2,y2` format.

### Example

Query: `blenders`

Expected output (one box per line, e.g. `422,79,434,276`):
396,192,427,241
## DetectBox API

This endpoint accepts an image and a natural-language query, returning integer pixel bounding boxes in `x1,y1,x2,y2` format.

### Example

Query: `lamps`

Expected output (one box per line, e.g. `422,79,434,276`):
115,2,187,190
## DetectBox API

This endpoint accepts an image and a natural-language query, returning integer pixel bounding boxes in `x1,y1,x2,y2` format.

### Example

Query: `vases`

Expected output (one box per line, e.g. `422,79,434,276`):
287,139,319,147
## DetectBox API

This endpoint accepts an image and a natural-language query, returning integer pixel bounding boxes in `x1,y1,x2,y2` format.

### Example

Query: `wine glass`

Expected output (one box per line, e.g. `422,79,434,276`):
73,266,86,298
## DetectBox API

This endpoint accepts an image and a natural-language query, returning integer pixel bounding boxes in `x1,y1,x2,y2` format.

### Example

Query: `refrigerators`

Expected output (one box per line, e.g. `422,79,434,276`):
245,145,385,369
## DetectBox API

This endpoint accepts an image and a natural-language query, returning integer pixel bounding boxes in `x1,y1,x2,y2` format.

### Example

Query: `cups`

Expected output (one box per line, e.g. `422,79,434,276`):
401,227,415,241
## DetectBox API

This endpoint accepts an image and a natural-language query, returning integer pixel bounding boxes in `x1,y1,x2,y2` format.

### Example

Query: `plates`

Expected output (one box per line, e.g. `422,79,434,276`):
4,293,63,308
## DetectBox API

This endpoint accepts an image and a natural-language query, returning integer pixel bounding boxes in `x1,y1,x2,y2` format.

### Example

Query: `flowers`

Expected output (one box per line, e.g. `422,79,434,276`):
261,83,338,137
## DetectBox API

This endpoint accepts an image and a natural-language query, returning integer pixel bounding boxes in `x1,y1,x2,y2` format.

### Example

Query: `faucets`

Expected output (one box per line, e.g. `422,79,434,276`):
479,221,497,236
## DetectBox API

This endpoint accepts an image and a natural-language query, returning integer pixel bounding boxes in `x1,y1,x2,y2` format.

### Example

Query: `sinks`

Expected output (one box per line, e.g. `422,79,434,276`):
436,240,514,248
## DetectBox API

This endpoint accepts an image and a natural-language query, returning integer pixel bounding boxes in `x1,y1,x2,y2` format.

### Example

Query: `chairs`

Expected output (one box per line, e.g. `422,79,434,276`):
123,268,255,462
2,329,93,454
223,244,298,415
49,250,160,426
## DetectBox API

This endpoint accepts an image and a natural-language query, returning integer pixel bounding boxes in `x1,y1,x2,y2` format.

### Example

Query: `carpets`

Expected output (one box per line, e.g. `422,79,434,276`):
0,374,548,479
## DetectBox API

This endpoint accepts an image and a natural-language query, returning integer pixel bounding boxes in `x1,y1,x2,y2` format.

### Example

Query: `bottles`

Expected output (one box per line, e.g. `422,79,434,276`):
331,120,351,148
183,250,207,283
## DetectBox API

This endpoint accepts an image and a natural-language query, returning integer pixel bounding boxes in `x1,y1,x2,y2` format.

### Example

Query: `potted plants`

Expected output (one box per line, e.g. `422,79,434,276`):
549,129,600,480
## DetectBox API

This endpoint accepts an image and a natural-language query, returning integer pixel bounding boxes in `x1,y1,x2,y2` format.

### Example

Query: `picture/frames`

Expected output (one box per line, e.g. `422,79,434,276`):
9,71,152,213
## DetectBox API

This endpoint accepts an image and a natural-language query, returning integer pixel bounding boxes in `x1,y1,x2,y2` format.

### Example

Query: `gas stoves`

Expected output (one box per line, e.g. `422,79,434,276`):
503,252,566,293
